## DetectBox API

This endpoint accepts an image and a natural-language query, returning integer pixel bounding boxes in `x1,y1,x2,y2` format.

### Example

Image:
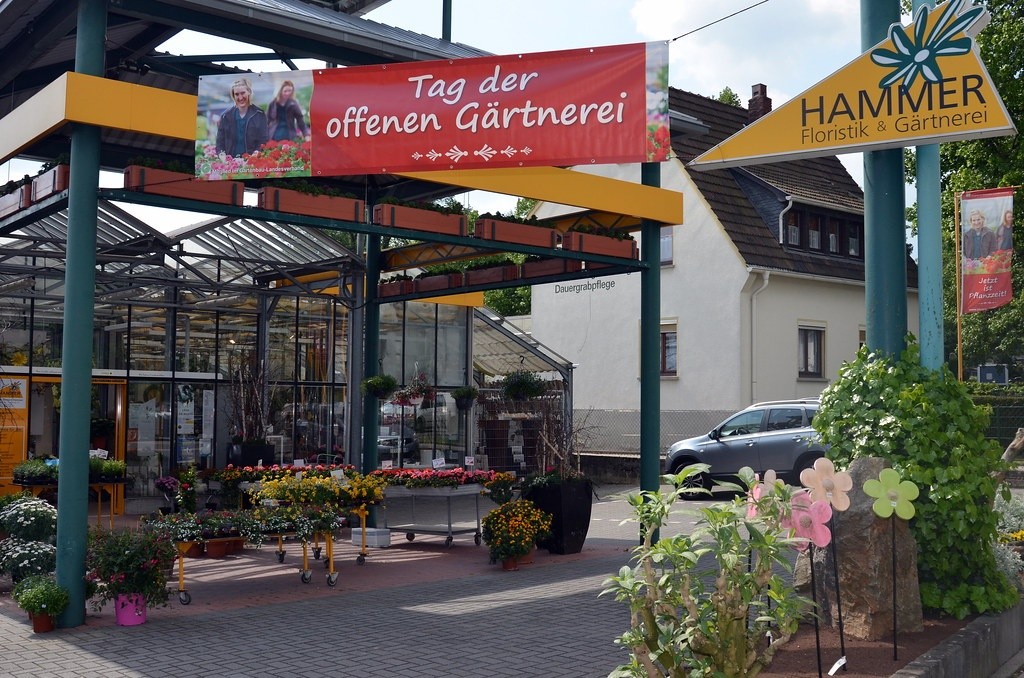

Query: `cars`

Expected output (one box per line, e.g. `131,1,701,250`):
281,401,420,460
126,443,163,465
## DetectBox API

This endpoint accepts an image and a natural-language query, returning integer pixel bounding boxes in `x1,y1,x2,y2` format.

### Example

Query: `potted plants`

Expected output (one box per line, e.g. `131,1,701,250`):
222,349,278,471
0,175,34,221
585,248,639,270
465,257,520,286
373,197,468,237
450,385,479,411
12,459,127,485
31,159,70,203
258,180,364,223
123,157,244,207
413,267,463,293
378,274,413,298
562,224,636,259
475,212,557,248
521,254,582,278
500,368,545,411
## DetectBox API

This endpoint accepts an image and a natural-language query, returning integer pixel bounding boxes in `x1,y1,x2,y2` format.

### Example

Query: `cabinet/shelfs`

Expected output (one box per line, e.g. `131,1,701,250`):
384,493,482,547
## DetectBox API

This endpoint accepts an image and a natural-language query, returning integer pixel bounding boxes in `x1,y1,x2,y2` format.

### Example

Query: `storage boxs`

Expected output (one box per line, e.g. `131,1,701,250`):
352,527,390,548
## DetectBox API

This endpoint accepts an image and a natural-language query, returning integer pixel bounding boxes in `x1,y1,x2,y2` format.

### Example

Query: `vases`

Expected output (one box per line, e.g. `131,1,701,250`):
114,593,147,626
188,542,204,559
28,610,35,623
502,557,519,571
227,538,244,554
174,541,188,558
12,571,21,584
31,613,54,633
205,537,227,559
521,488,592,554
519,546,536,564
386,483,481,496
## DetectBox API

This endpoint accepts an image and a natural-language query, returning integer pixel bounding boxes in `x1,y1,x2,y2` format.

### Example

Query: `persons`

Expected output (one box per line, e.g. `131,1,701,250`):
215,78,268,163
996,209,1013,251
963,210,997,262
266,80,311,144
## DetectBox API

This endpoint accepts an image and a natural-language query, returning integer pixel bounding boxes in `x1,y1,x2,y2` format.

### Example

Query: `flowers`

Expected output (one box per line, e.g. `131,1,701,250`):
480,472,515,505
369,467,495,489
519,466,592,487
0,497,70,618
481,500,553,546
487,514,537,564
11,347,100,409
148,463,388,552
195,129,311,181
85,525,179,612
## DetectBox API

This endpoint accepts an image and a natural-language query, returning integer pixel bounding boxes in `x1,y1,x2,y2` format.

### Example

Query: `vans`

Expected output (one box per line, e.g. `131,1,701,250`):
379,391,448,434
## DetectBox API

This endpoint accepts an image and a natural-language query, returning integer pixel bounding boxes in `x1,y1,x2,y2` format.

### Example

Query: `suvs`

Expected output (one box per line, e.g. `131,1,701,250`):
664,395,834,499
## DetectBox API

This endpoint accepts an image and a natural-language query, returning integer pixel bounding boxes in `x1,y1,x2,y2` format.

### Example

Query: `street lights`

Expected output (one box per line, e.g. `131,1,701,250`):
154,412,172,465
